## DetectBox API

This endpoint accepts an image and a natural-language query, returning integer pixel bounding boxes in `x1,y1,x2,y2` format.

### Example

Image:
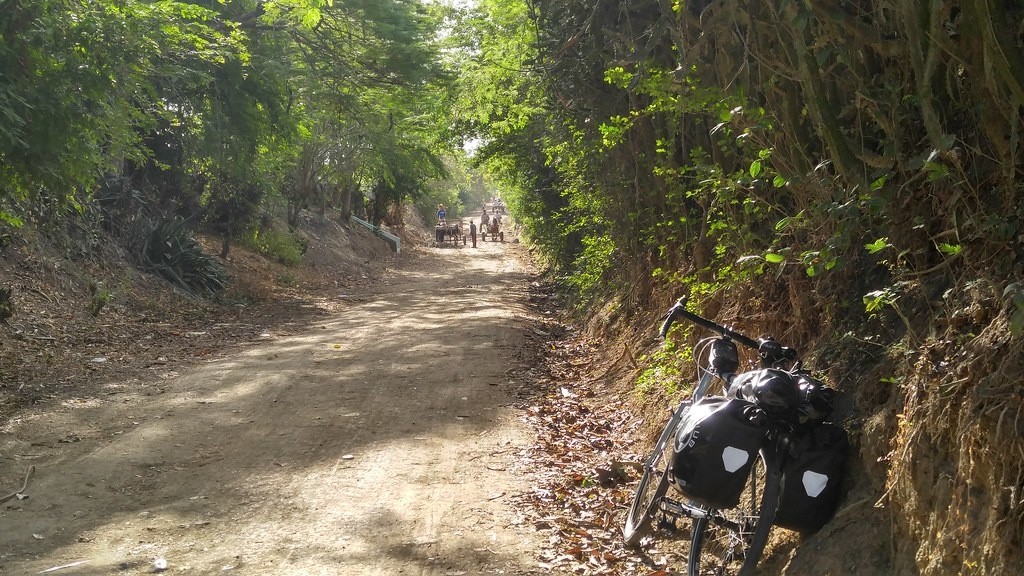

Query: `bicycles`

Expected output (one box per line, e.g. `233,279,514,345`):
621,293,812,576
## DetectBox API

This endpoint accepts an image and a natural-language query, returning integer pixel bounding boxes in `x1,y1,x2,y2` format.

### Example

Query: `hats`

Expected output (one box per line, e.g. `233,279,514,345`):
438,204,443,208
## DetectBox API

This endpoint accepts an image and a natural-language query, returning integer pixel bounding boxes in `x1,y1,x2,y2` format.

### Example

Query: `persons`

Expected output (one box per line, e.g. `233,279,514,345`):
436,204,446,225
479,197,502,233
469,220,477,248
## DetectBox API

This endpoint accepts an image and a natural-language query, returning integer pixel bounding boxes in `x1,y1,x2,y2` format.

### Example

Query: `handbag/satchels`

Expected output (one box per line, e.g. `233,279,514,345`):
767,423,846,534
671,396,765,508
